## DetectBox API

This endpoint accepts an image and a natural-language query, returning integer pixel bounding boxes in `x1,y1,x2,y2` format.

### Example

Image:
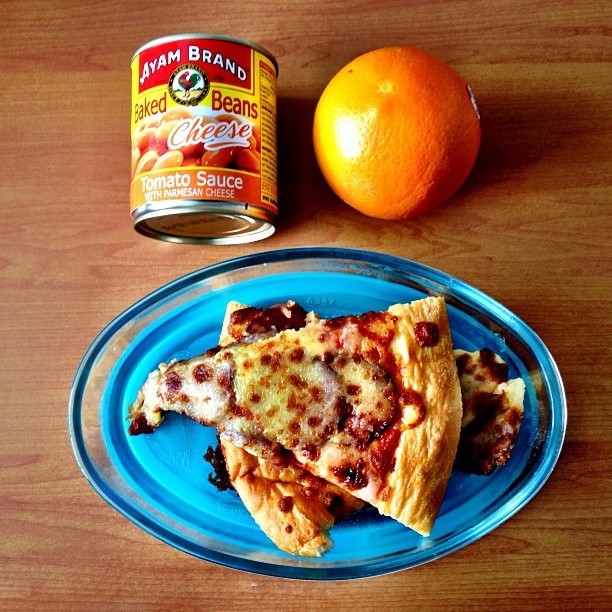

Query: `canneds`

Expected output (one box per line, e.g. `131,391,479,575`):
129,32,277,246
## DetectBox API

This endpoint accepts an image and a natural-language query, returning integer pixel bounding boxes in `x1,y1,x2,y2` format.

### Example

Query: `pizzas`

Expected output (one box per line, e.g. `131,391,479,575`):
123,296,464,535
218,301,513,556
202,375,534,492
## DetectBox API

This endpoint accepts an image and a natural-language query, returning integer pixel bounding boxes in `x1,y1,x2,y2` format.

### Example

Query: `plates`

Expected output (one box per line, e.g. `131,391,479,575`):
66,245,569,583
